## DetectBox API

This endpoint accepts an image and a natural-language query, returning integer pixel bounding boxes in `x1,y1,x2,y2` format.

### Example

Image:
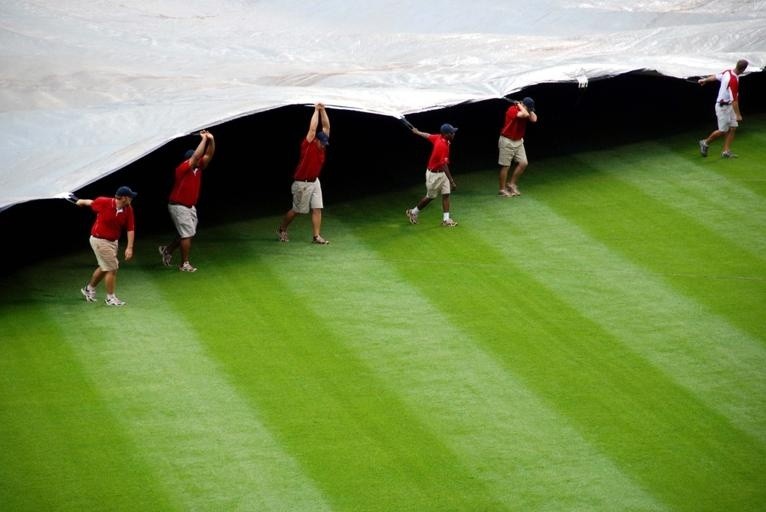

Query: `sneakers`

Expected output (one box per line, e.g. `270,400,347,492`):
80,285,97,304
722,151,736,159
697,138,708,157
276,230,289,242
406,209,417,223
496,190,512,197
312,235,328,244
158,245,172,266
178,261,198,273
441,219,457,227
104,295,126,306
507,183,520,196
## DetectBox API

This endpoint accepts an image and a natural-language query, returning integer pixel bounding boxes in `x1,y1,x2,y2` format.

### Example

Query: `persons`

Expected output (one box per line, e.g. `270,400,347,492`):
76,187,138,306
498,97,538,198
275,103,331,244
405,124,458,226
159,129,215,273
698,60,748,158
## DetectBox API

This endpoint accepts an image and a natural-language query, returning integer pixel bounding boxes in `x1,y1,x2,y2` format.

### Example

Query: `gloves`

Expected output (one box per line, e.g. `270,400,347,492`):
66,194,80,203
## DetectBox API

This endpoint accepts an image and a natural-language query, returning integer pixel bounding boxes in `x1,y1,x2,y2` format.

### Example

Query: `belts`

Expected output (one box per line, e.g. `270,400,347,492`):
169,202,192,208
294,178,317,182
718,101,730,106
426,168,443,174
500,134,512,140
92,235,115,242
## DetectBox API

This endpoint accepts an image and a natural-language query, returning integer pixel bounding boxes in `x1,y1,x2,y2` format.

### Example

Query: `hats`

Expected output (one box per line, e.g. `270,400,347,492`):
315,131,329,146
522,97,534,111
116,186,136,198
440,124,458,135
185,149,194,160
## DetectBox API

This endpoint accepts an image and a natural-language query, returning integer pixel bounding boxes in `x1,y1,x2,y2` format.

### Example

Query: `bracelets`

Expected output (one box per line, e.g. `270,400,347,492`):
704,78,707,82
